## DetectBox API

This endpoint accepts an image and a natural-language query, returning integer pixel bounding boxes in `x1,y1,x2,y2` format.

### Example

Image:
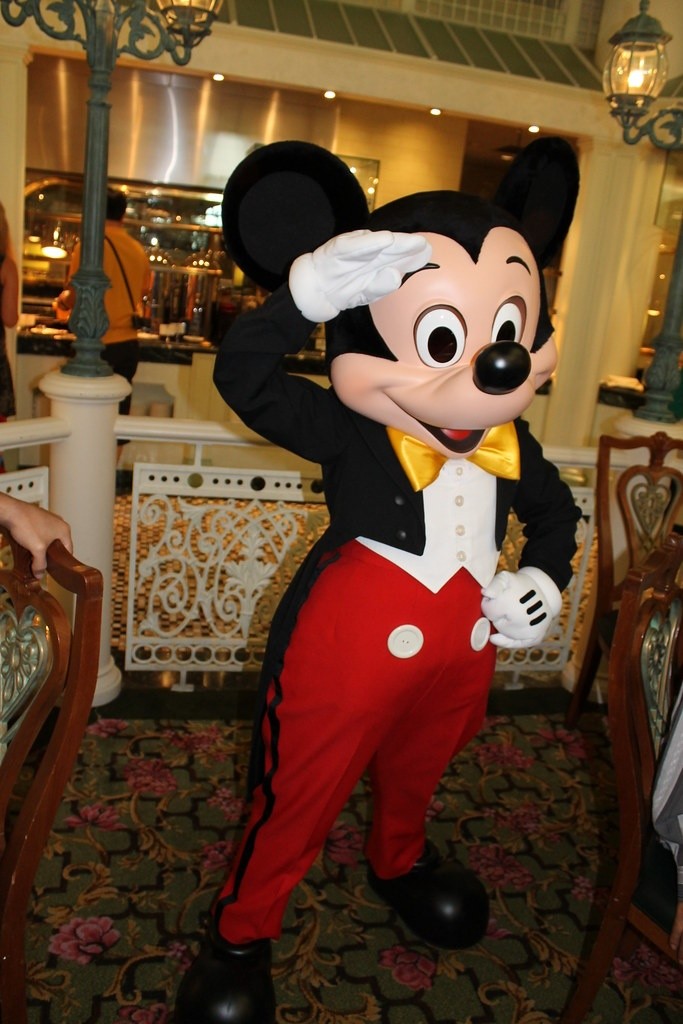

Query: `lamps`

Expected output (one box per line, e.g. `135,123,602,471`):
602,0,683,425
0,0,225,377
41,217,70,259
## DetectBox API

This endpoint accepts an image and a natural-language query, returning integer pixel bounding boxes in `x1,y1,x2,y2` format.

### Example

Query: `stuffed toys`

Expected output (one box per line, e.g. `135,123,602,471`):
189,135,582,1023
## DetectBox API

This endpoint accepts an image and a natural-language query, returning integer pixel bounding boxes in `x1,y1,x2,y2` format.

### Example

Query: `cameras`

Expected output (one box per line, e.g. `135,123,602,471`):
132,315,152,329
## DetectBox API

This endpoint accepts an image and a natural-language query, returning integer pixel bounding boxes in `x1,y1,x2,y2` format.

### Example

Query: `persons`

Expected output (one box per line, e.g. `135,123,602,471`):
0,203,19,422
57,188,150,466
1,492,74,575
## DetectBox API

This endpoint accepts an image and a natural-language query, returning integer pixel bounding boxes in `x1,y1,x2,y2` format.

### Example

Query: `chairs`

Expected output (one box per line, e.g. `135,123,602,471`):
562,431,683,725
558,532,683,1024
0,532,104,1024
